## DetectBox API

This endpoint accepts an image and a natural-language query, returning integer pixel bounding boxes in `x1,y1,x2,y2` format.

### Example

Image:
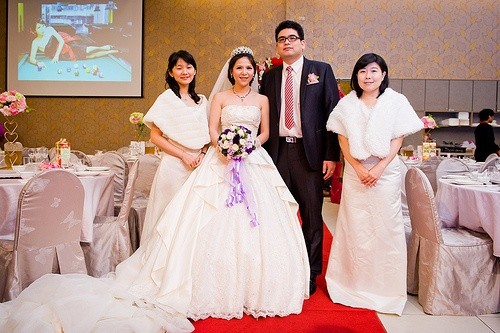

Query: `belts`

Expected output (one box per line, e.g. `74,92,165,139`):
279,136,303,144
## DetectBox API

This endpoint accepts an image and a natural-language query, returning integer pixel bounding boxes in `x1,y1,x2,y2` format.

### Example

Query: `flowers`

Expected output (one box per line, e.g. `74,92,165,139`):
130,112,145,126
306,72,319,85
0,91,31,117
420,115,439,129
217,124,256,160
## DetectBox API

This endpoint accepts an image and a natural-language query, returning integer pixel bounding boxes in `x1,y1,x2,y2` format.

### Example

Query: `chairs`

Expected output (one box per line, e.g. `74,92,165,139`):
0,152,500,318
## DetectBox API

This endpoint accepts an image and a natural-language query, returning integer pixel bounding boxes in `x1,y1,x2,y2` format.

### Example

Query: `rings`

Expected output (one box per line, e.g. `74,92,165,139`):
331,171,333,173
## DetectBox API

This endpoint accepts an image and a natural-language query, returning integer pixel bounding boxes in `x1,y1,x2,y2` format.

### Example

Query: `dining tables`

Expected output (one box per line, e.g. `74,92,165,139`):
0,165,116,242
435,172,500,258
398,155,483,171
123,152,162,170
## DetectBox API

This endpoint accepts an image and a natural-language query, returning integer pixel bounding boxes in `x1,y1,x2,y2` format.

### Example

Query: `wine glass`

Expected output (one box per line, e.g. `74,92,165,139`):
27,147,56,164
488,158,500,185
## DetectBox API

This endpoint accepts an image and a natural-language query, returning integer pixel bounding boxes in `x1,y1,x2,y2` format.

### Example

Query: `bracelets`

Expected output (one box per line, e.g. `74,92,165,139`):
202,151,206,154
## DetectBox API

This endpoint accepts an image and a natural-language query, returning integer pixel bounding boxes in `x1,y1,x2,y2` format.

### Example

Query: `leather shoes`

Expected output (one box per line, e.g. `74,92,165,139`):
310,272,317,294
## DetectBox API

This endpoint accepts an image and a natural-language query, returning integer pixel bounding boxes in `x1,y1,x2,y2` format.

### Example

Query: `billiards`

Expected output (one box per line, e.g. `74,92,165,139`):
38,63,103,78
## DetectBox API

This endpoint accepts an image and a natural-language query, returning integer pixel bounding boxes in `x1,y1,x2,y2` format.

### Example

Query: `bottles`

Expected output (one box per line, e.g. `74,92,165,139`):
56,138,71,169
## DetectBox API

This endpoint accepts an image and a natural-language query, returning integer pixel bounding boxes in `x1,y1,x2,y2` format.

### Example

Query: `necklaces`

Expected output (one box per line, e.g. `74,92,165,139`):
232,86,252,102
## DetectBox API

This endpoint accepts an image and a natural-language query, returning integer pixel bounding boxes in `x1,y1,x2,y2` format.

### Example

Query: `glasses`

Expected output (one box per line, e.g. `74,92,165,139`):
276,35,301,43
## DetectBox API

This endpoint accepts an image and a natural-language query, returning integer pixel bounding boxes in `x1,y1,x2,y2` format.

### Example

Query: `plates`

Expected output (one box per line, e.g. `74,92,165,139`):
75,166,110,176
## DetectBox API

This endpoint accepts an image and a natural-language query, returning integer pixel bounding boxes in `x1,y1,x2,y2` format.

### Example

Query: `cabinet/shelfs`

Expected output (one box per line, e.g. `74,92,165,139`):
388,79,500,152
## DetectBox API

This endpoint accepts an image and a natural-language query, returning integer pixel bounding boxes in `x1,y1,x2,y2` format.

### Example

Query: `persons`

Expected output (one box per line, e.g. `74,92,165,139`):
262,20,341,295
140,50,211,248
0,45,310,333
326,53,426,316
474,108,500,162
28,17,120,68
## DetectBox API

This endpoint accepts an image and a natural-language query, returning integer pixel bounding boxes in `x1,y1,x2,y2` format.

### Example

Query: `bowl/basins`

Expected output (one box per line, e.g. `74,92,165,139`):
12,165,35,178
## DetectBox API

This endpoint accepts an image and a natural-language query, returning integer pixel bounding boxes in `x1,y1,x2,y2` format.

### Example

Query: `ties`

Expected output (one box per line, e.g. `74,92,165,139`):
284,66,295,130
